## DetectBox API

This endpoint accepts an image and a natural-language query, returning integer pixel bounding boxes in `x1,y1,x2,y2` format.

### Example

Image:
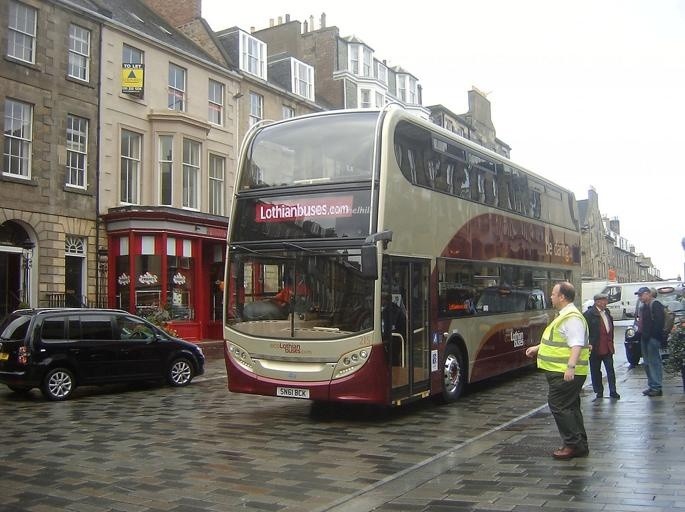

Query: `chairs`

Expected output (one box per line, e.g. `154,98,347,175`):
394,136,540,221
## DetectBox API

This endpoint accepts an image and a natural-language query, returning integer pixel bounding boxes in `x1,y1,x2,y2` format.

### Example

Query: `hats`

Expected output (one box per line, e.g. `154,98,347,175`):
594,293,608,300
634,287,650,295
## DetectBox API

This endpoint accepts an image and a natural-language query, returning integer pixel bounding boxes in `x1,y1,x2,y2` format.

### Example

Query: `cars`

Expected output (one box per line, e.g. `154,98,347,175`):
438,282,475,318
0,305,207,400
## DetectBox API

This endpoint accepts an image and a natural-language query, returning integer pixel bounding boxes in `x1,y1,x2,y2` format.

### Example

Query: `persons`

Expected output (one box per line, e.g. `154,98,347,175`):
268,268,308,309
525,282,589,458
378,292,405,367
117,319,133,339
582,294,620,399
634,286,666,397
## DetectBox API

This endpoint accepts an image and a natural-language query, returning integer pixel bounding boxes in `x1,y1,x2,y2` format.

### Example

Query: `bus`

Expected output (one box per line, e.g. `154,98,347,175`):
221,100,587,417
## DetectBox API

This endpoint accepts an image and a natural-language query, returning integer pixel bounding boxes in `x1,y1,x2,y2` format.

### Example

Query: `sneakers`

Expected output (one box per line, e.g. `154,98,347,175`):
643,389,662,396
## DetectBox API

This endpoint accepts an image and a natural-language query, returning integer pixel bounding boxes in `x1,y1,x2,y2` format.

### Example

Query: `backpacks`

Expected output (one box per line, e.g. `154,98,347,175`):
650,300,675,343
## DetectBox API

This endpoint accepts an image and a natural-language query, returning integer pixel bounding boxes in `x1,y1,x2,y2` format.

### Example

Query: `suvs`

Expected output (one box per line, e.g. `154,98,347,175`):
624,284,685,364
472,285,546,313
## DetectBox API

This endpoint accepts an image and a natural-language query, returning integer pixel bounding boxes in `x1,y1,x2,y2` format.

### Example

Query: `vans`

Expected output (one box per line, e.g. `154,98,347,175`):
583,281,685,320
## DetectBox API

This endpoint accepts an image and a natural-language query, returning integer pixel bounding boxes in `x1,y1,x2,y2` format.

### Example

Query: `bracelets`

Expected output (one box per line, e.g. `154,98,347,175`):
567,363,576,368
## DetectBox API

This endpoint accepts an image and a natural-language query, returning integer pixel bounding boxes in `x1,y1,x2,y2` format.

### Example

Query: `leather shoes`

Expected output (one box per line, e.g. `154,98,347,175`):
598,392,603,397
552,443,587,460
610,392,620,398
